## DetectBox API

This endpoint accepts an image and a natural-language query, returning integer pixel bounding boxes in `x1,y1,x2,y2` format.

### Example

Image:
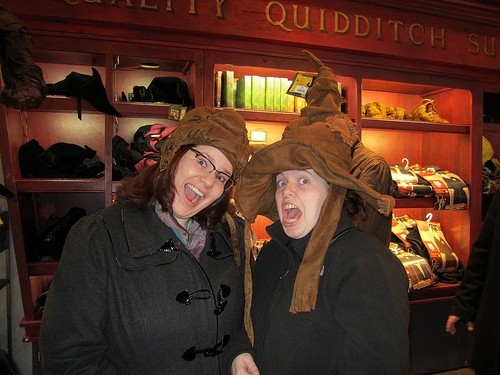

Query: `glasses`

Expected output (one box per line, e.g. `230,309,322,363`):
188,146,237,192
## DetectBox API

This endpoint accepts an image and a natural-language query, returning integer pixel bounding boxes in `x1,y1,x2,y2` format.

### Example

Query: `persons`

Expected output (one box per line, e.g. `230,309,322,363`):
445,183,500,375
225,50,410,375
39,106,255,375
481,158,500,221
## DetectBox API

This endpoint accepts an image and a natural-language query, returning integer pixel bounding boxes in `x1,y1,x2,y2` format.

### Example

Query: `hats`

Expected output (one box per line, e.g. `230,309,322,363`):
231,50,398,225
158,105,253,179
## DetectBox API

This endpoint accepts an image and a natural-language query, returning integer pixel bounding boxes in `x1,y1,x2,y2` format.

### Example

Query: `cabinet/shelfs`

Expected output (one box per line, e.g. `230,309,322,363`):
0,0,500,375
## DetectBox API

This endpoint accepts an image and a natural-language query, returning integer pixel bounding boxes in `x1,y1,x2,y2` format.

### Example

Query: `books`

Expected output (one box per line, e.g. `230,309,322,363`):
214,71,342,113
